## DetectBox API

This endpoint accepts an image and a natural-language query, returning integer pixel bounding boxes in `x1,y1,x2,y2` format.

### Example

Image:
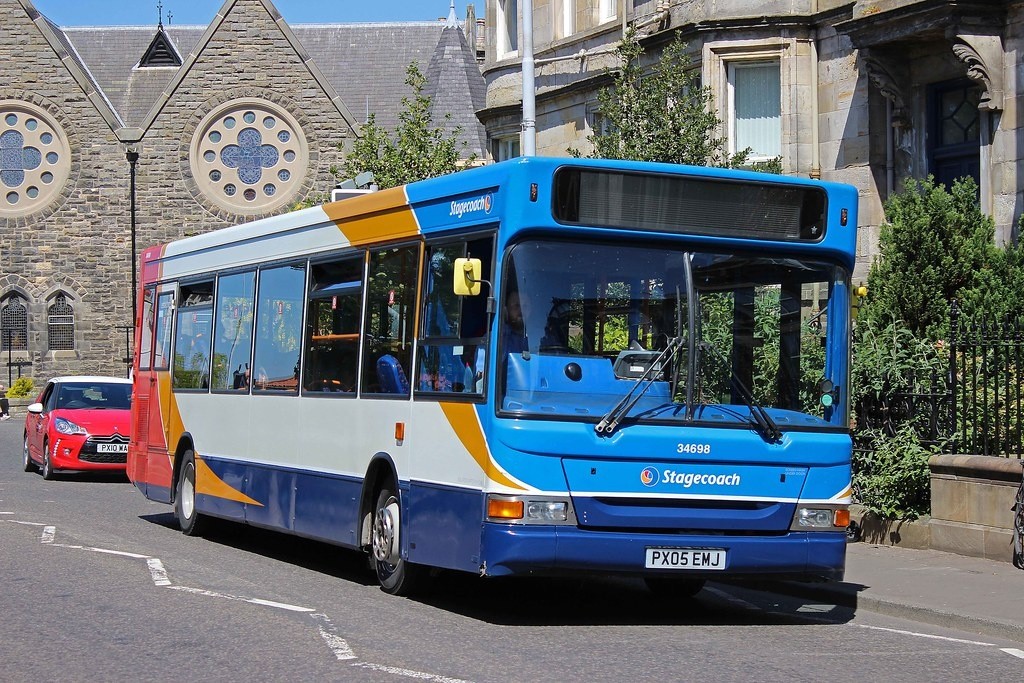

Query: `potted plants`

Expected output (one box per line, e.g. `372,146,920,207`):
3,374,37,418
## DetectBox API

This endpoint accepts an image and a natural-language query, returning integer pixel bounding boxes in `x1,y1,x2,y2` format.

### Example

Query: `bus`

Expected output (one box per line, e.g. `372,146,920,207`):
123,153,867,599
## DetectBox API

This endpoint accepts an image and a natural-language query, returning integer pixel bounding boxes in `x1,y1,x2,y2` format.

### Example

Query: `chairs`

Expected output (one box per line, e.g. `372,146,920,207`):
376,353,410,394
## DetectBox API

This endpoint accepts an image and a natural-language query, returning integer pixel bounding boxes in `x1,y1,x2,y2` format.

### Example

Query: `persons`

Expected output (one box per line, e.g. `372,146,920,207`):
244,366,268,390
0,385,10,420
475,289,562,394
71,390,82,400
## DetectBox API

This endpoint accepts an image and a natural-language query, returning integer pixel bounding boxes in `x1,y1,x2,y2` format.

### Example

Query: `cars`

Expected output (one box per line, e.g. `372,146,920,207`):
22,374,136,480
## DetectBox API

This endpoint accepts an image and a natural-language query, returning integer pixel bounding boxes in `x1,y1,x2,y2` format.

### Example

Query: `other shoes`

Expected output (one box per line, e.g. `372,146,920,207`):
0,414,11,421
